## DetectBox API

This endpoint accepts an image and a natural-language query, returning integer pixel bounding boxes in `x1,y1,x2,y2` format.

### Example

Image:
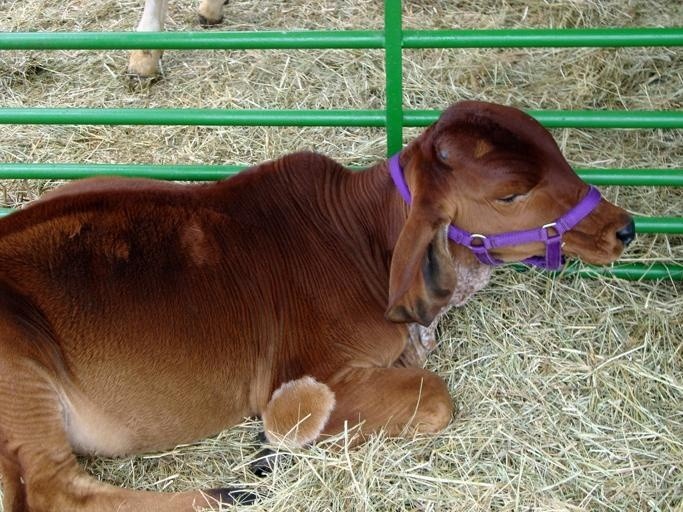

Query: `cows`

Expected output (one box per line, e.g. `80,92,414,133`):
0,98,639,512
126,0,231,94
